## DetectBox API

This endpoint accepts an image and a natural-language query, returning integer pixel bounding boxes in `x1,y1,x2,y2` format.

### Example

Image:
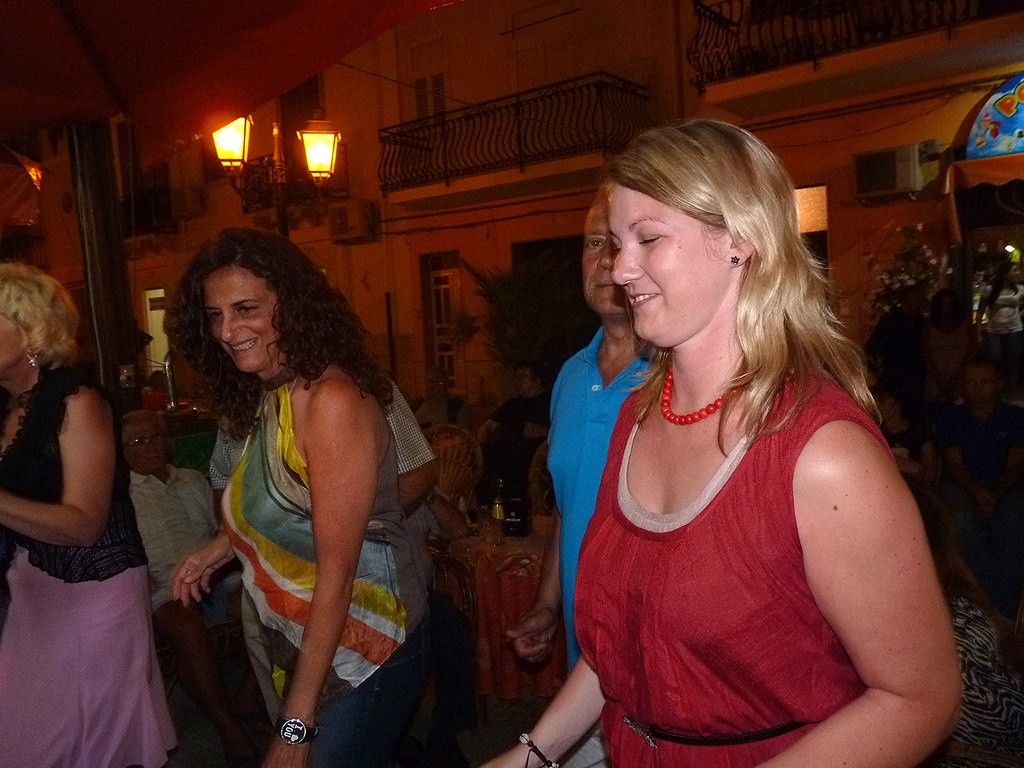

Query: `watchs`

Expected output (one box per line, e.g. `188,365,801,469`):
274,716,316,745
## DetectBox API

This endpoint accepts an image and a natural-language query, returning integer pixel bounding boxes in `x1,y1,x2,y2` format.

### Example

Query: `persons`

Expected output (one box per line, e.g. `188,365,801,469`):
976,261,1024,398
863,281,969,490
477,358,551,497
395,483,477,768
900,470,1024,768
122,409,262,768
165,226,435,768
0,263,176,768
505,180,671,768
142,370,170,410
869,354,1024,619
471,120,964,768
409,367,466,430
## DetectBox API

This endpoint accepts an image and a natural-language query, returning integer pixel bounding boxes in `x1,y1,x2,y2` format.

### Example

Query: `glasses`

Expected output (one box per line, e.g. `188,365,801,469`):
121,433,168,449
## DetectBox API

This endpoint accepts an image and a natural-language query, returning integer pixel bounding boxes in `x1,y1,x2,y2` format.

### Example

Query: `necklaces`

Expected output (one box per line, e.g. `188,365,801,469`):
661,364,742,426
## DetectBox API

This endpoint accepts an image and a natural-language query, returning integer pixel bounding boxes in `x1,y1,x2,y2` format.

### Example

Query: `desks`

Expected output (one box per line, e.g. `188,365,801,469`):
435,511,570,700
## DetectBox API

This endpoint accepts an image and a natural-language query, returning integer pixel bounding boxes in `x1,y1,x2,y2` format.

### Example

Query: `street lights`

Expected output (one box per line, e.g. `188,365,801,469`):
209,115,342,240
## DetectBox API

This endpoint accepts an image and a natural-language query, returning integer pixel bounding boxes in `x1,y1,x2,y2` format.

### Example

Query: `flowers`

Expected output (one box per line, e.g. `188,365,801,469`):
860,219,953,323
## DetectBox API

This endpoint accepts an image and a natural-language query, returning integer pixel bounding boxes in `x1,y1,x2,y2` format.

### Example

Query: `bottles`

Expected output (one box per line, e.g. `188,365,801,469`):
119,348,138,389
490,479,506,520
477,504,504,545
435,365,449,394
918,139,941,184
505,498,529,536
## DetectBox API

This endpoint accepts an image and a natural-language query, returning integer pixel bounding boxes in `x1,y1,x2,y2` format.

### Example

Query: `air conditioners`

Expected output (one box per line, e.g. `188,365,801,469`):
853,143,923,199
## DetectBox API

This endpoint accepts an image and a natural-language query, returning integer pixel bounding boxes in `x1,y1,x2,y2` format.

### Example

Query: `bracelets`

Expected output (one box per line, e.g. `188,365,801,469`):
519,733,560,768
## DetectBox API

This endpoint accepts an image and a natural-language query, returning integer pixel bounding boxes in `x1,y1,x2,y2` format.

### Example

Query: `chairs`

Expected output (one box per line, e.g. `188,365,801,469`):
529,441,555,516
422,423,487,734
167,414,218,478
154,617,247,690
